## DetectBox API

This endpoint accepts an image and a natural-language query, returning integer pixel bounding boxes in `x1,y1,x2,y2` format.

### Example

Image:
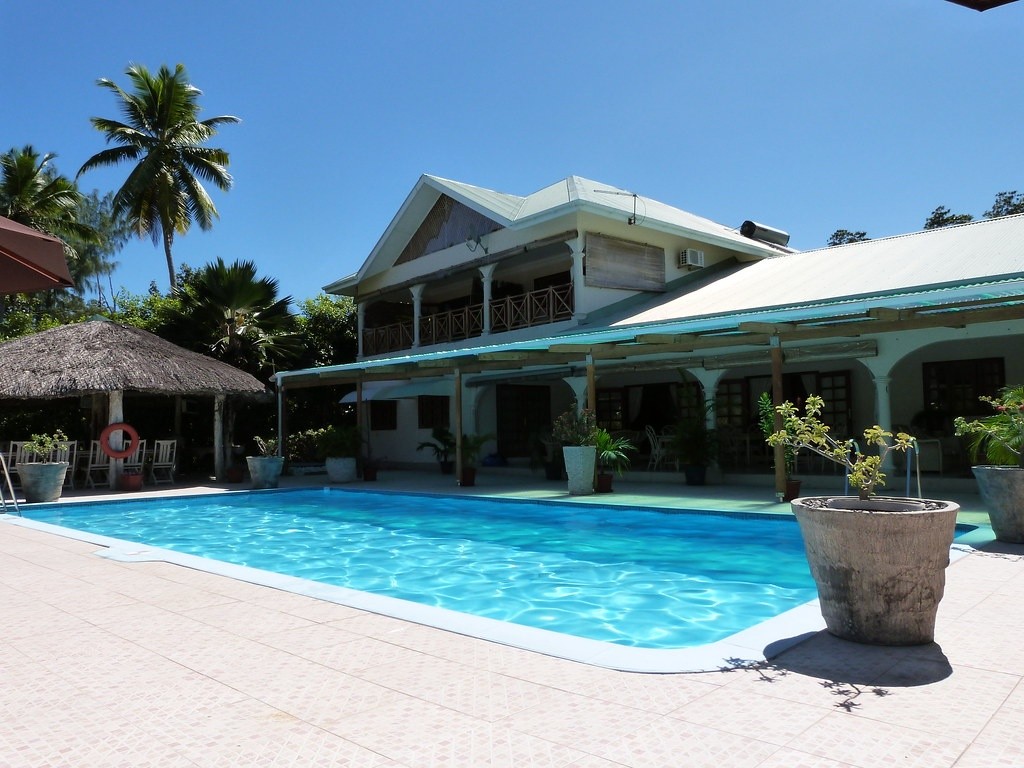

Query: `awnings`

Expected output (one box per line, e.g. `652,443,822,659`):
0,216,75,296
265,275,1024,501
339,379,453,403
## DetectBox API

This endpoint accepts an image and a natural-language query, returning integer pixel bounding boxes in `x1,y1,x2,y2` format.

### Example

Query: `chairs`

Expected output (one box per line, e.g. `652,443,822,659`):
122,441,148,488
79,440,110,490
645,425,680,472
1,440,40,494
48,440,78,490
145,439,178,486
900,437,954,477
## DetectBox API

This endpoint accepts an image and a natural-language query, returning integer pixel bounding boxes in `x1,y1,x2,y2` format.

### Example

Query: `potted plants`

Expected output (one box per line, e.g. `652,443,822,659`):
461,432,496,486
245,435,284,488
320,427,361,483
954,383,1024,543
672,394,742,485
595,427,638,491
538,434,565,480
765,394,961,646
758,392,801,501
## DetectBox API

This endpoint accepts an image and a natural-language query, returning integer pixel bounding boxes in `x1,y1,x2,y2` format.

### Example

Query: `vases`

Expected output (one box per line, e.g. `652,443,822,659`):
122,473,145,491
563,446,596,495
15,461,70,501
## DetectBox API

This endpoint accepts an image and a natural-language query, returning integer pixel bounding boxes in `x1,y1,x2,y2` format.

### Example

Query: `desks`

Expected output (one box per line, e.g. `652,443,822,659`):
935,436,984,474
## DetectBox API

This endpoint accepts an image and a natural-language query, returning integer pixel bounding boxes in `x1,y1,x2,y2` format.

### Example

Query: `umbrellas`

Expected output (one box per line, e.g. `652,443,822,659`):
0,321,266,397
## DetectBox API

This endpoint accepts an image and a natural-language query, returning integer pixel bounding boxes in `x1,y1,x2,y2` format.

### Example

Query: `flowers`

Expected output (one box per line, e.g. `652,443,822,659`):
552,401,601,446
19,429,68,463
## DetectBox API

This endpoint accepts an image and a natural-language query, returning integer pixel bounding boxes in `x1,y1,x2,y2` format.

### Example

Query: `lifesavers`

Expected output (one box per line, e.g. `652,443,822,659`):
99,422,139,459
